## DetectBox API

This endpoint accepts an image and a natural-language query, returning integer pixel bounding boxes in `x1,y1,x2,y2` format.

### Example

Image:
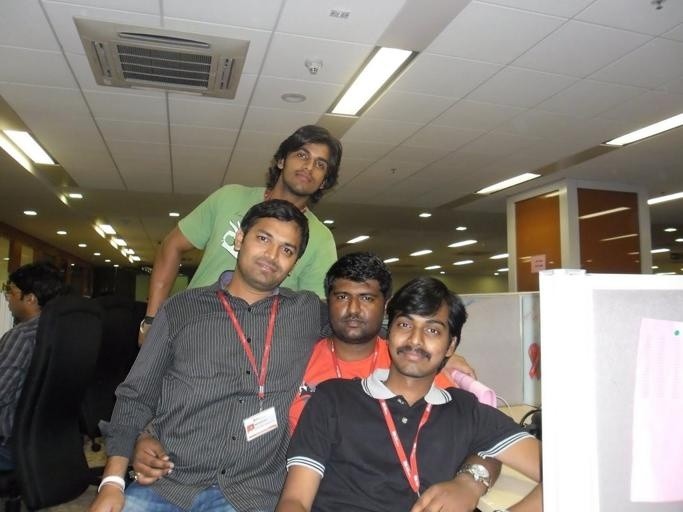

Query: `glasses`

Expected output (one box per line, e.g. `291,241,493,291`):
4,292,21,302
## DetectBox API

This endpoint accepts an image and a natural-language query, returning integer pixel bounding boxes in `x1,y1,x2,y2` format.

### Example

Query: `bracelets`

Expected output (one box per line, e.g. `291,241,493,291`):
95,476,127,491
138,315,156,327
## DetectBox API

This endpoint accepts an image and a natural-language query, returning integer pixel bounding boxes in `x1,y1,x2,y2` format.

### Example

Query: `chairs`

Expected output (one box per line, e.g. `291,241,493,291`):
0,293,148,512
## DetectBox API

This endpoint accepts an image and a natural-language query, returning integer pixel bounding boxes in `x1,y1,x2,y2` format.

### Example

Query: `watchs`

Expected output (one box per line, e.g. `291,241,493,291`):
457,464,493,494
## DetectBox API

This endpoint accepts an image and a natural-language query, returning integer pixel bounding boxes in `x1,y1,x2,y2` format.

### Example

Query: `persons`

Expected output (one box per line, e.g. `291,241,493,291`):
136,122,343,347
288,253,503,512
274,278,542,512
89,199,476,511
1,263,62,470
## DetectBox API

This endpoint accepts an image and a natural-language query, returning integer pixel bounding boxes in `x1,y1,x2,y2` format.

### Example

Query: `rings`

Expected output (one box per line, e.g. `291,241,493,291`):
130,472,138,481
